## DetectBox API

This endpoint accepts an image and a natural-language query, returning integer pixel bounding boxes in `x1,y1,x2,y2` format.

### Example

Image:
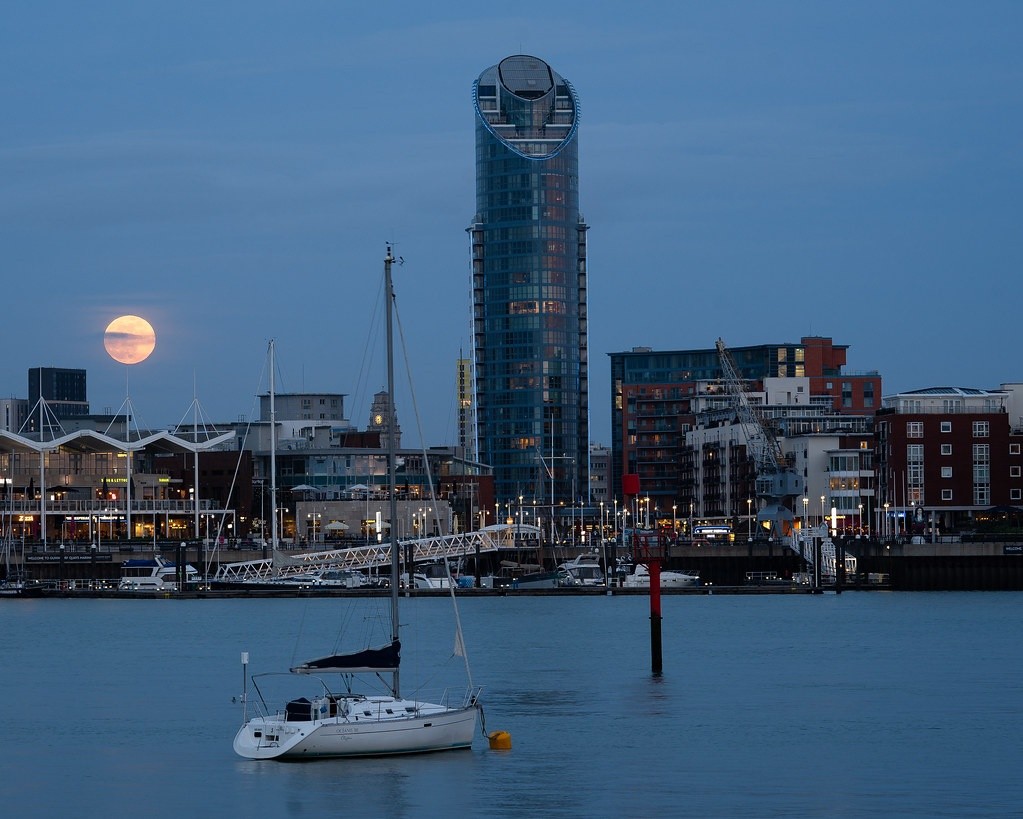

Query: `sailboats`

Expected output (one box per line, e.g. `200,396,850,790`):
231,236,484,762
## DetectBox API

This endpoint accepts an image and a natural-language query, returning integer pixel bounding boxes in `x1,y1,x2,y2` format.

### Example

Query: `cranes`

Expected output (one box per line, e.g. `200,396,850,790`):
713,335,805,545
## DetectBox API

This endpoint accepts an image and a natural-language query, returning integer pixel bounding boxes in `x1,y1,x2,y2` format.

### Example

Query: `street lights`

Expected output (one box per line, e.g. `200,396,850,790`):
639,507,644,529
307,506,321,541
636,499,639,528
599,501,604,546
820,495,825,522
746,499,752,542
275,501,289,541
690,504,694,542
418,500,432,539
519,496,524,540
884,503,889,537
494,503,500,549
613,500,618,540
803,498,809,529
672,505,677,546
478,504,489,527
858,504,863,537
532,500,537,530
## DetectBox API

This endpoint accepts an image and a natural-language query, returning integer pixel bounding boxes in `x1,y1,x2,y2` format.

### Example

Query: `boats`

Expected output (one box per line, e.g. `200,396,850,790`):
116,549,701,592
